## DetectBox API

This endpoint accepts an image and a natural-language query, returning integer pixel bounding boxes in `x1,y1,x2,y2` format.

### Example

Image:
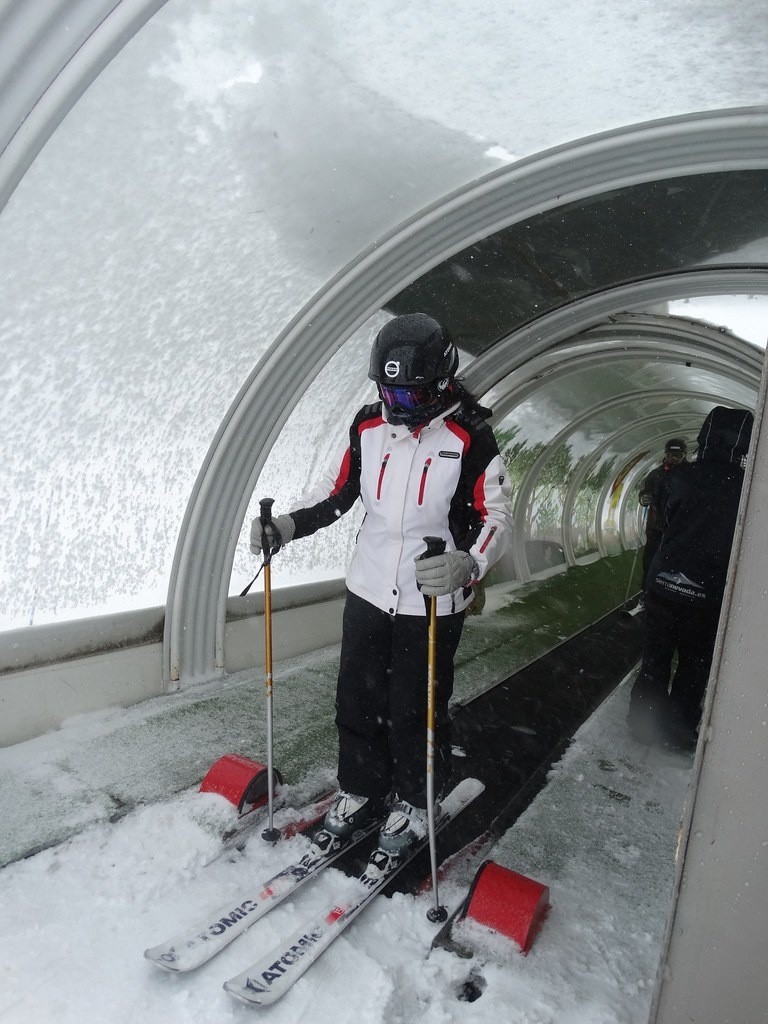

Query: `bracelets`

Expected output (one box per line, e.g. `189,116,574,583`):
463,558,480,588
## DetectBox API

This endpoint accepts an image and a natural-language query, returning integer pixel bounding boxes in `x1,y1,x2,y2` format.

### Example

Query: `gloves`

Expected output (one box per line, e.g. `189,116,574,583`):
415,549,478,597
249,516,295,555
639,493,652,507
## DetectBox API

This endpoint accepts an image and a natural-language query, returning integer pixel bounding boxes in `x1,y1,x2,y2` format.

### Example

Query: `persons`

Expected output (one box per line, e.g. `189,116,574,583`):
628,405,754,753
251,312,513,854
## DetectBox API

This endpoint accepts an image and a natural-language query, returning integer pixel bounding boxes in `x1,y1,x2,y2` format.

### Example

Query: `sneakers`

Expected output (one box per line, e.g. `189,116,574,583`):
380,795,442,848
326,785,387,838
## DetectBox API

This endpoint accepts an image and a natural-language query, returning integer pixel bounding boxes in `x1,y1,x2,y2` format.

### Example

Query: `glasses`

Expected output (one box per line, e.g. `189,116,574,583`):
665,451,686,461
379,383,436,412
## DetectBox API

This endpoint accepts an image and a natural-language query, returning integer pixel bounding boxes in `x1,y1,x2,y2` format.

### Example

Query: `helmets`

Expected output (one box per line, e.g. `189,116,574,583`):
368,313,458,427
665,438,687,456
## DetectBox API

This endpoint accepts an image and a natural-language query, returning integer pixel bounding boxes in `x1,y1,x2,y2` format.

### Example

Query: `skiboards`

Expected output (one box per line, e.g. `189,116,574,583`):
137,771,489,1009
619,603,646,619
